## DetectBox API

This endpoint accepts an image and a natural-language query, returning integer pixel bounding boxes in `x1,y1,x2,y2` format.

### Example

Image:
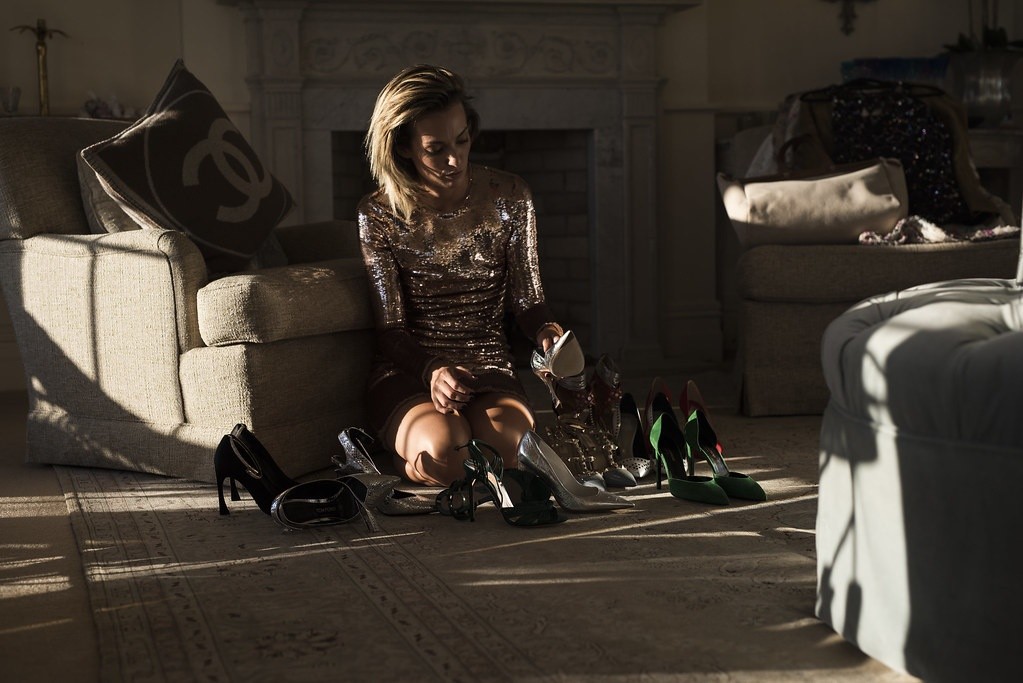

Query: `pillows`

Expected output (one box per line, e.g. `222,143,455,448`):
75,55,299,257
716,163,903,243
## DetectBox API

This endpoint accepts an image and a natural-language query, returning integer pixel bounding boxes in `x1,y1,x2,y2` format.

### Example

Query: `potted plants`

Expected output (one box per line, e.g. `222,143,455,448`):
942,26,1023,128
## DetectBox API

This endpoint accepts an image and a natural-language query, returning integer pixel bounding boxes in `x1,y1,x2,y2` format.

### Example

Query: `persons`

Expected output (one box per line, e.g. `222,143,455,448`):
359,62,564,487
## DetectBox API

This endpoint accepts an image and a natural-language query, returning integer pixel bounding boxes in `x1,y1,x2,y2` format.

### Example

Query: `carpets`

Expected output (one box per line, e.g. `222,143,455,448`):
52,403,919,683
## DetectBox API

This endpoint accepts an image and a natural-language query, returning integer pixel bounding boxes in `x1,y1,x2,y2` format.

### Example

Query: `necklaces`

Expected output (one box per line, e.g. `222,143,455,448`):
423,160,472,220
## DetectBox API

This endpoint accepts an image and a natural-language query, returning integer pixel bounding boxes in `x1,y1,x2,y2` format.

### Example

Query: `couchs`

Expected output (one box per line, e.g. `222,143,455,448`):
815,278,1023,683
720,123,1023,418
0,116,379,490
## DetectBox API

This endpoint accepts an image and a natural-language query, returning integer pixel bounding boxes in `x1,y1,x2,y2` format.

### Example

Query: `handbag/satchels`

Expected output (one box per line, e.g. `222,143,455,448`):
775,78,991,225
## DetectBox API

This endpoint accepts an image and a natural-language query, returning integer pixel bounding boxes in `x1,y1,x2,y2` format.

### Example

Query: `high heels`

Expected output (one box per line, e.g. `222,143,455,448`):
679,380,726,456
330,428,437,515
435,439,569,526
271,472,402,534
517,328,654,512
644,376,672,459
650,413,731,507
215,425,300,515
686,409,767,502
